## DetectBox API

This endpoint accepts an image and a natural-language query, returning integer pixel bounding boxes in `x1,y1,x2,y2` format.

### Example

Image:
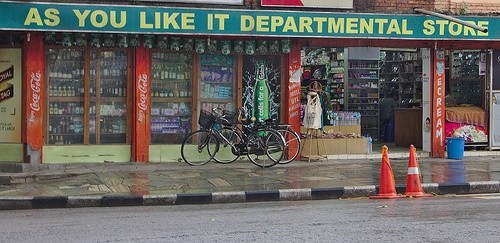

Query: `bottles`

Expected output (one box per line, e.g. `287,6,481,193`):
347,60,379,68
149,51,192,98
330,111,361,126
43,47,128,145
252,63,272,137
362,134,373,153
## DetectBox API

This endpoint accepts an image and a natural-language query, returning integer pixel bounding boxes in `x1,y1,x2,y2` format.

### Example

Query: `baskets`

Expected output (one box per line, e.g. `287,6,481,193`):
198,109,216,128
221,110,236,125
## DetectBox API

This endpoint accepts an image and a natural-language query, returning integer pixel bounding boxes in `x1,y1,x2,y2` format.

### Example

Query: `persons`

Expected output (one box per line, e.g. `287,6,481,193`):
424,117,431,133
381,91,395,142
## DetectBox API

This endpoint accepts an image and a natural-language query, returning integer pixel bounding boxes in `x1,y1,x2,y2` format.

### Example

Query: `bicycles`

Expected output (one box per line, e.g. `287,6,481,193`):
181,105,302,169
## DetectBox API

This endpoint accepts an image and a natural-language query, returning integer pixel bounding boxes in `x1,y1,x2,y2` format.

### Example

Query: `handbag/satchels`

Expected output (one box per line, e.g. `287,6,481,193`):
303,93,322,129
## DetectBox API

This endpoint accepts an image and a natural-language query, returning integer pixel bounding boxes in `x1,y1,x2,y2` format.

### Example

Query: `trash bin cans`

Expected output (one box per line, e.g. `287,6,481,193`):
446,138,465,158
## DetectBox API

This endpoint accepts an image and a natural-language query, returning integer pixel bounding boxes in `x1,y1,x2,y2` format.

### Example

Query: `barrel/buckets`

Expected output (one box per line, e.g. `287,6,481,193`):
446,136,465,159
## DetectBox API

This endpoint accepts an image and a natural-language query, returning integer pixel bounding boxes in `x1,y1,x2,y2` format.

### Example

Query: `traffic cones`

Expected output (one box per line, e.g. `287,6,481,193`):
401,144,433,197
369,145,407,199
